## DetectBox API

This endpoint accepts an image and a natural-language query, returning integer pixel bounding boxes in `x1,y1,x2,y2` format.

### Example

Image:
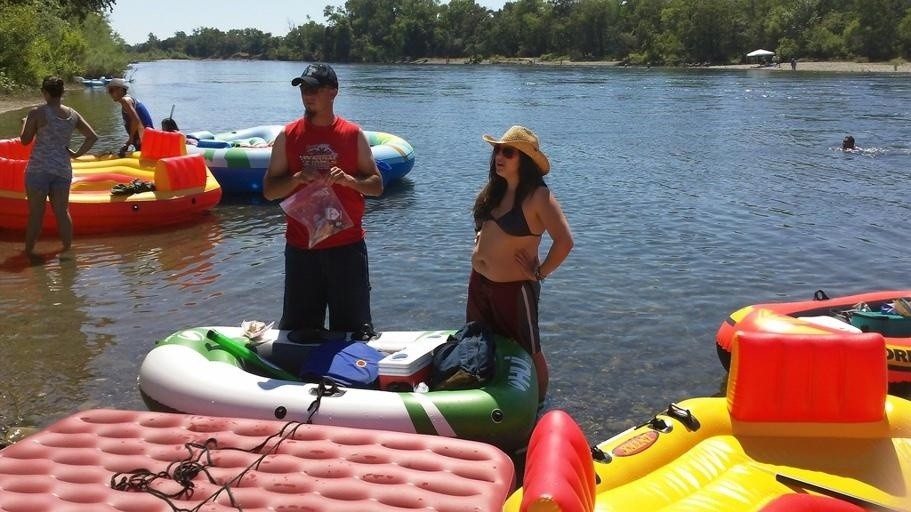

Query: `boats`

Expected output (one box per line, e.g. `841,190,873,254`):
136,324,538,445
715,289,911,397
500,334,911,511
0,411,516,511
182,125,415,199
83,77,133,88
0,133,228,234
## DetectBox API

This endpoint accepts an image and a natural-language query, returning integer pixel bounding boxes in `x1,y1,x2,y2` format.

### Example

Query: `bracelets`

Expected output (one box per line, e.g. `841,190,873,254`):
535,266,547,285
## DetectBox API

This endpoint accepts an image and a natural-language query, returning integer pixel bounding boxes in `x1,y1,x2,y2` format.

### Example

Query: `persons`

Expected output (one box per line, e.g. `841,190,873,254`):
840,136,862,151
105,78,154,152
19,75,98,255
262,62,385,332
466,124,574,410
791,58,796,70
792,61,797,71
162,118,178,132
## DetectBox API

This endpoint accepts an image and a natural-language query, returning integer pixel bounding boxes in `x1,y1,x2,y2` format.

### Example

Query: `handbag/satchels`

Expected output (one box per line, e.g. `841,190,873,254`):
428,320,495,391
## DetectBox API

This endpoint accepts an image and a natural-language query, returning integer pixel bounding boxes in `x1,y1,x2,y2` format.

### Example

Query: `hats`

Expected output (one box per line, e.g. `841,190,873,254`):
482,126,550,176
106,77,129,90
292,63,339,89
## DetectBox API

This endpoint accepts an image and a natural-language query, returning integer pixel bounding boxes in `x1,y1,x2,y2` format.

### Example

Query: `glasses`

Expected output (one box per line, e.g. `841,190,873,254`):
492,145,519,160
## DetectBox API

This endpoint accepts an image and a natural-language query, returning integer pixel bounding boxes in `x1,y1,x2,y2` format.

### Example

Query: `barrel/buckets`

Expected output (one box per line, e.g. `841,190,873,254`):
852,307,904,338
